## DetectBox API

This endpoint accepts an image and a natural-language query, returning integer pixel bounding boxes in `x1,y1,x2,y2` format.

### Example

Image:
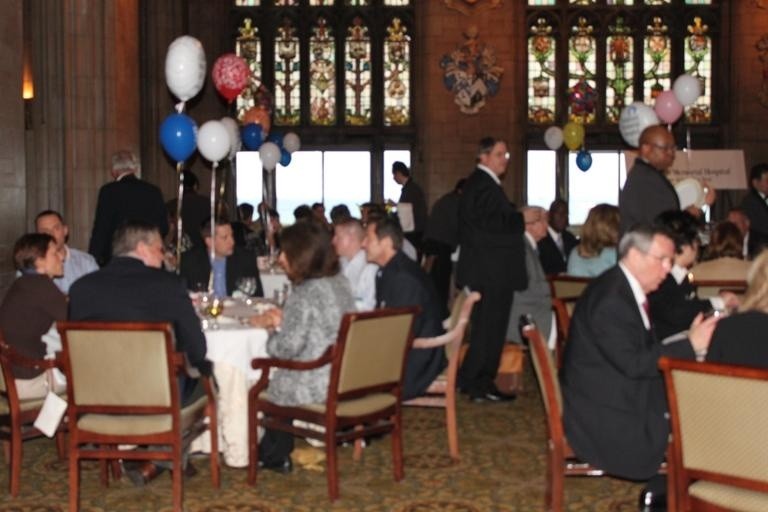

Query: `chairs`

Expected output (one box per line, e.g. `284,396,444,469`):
551,295,580,336
249,306,420,499
657,355,768,512
0,337,123,495
352,286,481,459
544,275,591,297
458,339,529,395
56,321,220,512
518,313,668,512
693,280,748,299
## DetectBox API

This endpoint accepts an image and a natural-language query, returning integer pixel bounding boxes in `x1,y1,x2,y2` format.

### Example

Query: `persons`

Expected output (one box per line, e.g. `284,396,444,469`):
503,200,767,511
392,162,428,248
86,150,168,266
425,179,463,266
740,163,767,256
165,171,219,251
453,136,540,401
619,125,716,237
0,201,443,485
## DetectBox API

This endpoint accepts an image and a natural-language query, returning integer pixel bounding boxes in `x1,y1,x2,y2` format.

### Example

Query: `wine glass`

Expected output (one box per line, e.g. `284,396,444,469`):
210,294,224,331
241,276,256,305
198,294,212,329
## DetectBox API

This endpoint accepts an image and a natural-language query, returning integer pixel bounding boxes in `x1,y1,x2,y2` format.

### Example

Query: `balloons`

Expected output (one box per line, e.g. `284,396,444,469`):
618,101,659,148
159,35,300,171
673,74,702,107
655,91,683,124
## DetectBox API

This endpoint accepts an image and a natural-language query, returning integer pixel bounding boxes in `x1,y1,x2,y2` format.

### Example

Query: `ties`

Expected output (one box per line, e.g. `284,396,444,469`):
643,296,655,336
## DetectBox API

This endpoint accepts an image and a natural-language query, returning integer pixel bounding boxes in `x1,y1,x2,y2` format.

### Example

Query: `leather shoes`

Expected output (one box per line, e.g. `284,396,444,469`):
460,386,493,404
257,455,293,474
169,464,199,483
128,462,158,486
485,386,517,402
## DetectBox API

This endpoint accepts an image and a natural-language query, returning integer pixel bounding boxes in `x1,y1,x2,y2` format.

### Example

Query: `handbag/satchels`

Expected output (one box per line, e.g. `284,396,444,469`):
33,390,68,440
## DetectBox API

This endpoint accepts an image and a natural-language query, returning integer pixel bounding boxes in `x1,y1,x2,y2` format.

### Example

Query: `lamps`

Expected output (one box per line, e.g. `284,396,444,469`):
23,97,34,130
